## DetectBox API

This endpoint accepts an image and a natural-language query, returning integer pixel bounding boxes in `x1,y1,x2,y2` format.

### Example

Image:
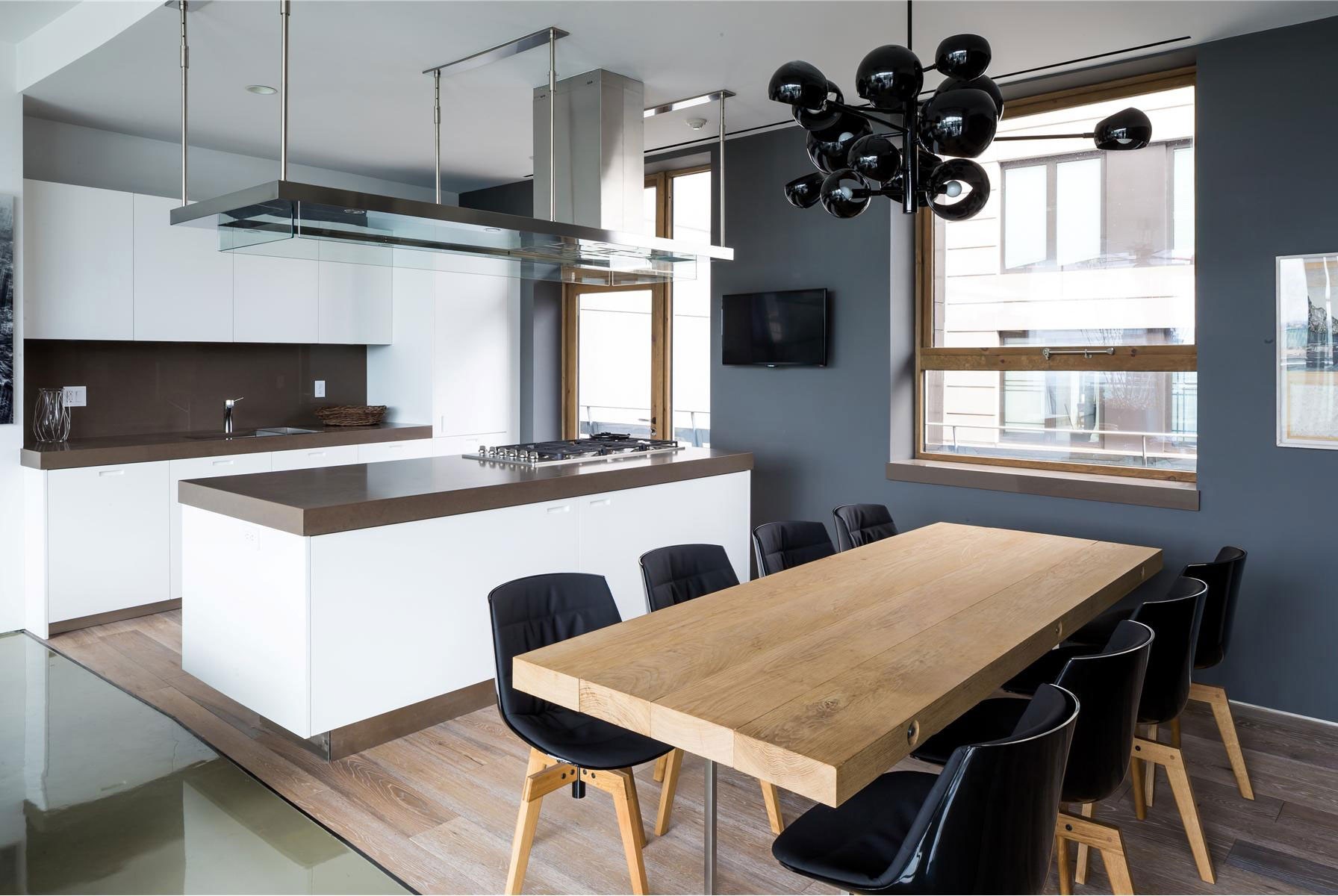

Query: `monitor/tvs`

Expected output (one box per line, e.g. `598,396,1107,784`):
722,288,828,368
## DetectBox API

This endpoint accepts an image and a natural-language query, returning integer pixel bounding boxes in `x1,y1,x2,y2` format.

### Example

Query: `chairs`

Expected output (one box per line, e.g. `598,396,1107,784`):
771,681,1081,895
1103,544,1256,807
485,571,685,895
750,519,836,579
636,543,791,839
1000,574,1218,884
832,502,898,553
907,615,1155,896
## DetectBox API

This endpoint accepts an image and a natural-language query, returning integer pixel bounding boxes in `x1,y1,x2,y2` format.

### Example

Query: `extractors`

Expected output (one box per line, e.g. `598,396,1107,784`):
509,67,734,287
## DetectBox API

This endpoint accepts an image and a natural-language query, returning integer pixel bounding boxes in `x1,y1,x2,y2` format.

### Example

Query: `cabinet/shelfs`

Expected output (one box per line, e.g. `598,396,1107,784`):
19,458,170,639
359,438,432,465
24,178,233,345
169,451,272,615
272,443,358,472
225,252,394,347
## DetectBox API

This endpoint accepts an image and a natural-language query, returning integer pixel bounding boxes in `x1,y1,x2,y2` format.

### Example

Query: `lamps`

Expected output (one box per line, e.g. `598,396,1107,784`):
766,1,1153,223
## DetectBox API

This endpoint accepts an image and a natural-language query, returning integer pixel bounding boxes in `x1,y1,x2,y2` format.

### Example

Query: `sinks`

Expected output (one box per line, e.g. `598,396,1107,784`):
185,430,284,443
254,426,326,437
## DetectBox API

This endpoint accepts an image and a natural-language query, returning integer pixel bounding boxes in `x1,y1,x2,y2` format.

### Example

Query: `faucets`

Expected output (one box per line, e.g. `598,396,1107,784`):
224,397,245,435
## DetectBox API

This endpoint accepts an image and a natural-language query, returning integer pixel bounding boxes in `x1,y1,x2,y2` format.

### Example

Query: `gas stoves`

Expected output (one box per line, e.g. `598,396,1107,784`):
462,431,686,468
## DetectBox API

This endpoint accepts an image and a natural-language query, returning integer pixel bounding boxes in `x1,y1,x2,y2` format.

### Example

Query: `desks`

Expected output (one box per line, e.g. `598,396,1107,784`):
512,521,1166,895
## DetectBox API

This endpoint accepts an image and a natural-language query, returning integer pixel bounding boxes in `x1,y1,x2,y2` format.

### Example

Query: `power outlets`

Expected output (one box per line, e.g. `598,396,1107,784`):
62,386,87,407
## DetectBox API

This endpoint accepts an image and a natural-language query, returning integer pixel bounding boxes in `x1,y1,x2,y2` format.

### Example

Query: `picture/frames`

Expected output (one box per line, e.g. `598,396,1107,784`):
1272,251,1338,451
